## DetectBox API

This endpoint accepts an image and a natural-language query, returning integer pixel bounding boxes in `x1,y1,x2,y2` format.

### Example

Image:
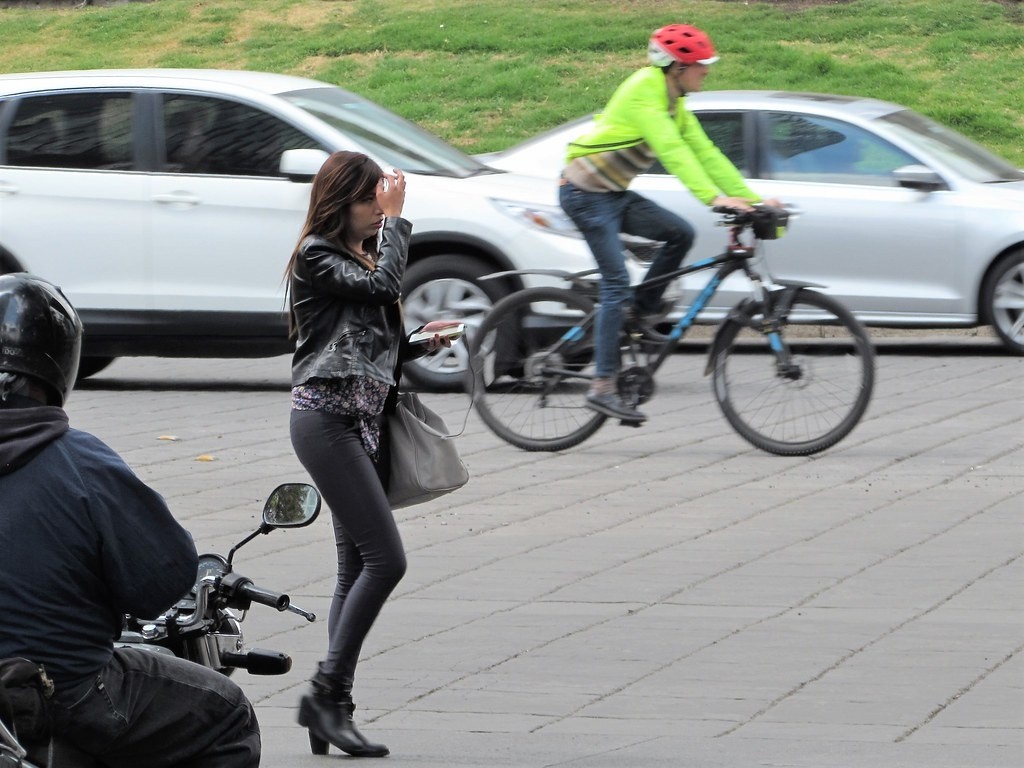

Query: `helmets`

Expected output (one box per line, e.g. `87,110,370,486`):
647,24,719,68
0,274,84,406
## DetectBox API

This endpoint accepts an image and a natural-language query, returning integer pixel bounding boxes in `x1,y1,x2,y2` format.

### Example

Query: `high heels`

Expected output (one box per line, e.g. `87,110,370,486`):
298,678,390,758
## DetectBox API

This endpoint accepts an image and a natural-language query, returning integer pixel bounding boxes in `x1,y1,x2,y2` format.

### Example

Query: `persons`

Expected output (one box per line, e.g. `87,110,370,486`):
282,151,460,757
559,24,777,418
1,273,261,768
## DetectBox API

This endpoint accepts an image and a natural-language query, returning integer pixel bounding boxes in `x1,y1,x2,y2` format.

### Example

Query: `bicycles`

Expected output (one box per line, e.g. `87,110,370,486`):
467,201,876,457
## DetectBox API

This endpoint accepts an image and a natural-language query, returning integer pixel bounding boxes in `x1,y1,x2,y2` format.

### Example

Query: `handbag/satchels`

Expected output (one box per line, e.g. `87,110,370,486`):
387,393,470,508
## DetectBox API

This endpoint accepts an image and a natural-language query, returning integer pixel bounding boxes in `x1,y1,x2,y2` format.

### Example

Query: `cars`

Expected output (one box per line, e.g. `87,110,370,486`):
0,66,621,391
463,91,1024,379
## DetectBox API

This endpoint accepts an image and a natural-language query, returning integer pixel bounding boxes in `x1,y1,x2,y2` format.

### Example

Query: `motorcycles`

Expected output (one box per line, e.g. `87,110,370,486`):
0,482,323,768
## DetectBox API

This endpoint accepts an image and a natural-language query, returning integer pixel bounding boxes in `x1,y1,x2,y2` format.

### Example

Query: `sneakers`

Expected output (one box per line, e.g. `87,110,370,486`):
586,389,645,423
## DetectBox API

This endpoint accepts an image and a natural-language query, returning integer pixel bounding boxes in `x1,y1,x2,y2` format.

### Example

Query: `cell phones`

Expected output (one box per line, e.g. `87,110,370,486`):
409,323,465,345
